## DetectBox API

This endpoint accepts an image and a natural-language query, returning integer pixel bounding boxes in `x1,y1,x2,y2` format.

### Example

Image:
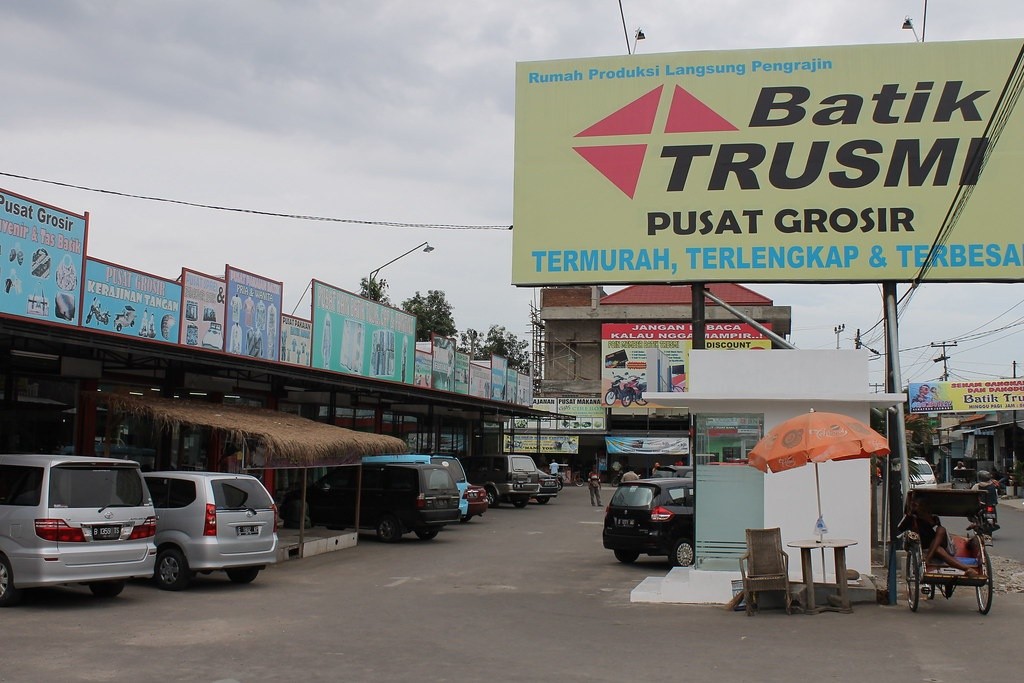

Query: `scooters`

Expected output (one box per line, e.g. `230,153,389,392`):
605,371,648,407
975,478,1007,538
86,297,111,326
114,305,135,332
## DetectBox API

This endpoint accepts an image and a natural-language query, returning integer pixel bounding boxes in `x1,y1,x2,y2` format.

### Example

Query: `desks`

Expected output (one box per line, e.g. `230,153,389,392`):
787,539,858,615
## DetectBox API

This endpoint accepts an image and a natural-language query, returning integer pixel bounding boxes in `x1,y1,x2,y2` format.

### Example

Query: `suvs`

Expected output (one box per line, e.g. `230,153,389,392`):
142,472,279,591
457,455,539,508
0,454,157,607
279,453,468,543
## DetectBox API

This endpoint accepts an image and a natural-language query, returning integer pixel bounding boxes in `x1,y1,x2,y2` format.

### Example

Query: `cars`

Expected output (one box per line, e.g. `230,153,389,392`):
460,482,488,522
536,469,558,503
603,466,694,570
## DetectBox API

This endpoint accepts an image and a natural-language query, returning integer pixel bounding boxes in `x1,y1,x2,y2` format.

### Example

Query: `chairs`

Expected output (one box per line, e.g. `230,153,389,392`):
738,527,792,616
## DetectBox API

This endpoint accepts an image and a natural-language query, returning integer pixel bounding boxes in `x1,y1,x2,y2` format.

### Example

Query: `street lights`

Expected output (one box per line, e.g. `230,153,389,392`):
368,242,434,302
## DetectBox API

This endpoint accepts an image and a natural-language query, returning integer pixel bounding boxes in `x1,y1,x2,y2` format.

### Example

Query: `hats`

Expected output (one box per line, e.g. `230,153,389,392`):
591,465,598,469
976,469,990,482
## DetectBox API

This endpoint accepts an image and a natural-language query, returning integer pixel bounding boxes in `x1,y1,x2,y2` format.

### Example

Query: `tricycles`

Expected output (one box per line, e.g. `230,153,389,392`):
902,488,993,615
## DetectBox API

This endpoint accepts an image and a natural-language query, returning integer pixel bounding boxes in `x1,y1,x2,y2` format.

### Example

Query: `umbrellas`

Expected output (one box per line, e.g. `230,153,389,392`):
748,407,892,602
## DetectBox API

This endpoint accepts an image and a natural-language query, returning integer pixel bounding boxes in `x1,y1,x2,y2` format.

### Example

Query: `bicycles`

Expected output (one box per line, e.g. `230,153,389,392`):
563,472,585,487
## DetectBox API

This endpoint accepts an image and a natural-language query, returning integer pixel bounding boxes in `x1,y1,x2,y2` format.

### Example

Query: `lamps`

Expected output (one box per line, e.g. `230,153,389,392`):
632,26,645,55
209,374,237,383
283,384,305,392
901,14,919,42
10,348,60,361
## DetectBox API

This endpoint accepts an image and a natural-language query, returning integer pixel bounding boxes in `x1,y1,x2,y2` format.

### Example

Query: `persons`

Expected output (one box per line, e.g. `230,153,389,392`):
937,458,999,529
898,500,978,575
621,466,639,483
588,466,603,506
549,459,559,476
677,457,687,466
652,463,660,474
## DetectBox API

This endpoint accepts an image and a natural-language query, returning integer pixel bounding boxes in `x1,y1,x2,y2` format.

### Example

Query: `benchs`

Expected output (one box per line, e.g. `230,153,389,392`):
923,534,978,571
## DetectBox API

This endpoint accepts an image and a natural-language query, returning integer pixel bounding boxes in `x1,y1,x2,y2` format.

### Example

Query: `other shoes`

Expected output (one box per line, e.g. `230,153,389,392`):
598,503,603,506
592,503,596,506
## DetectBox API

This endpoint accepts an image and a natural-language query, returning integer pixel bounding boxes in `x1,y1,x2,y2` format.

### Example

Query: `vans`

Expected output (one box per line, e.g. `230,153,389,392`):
897,459,937,494
